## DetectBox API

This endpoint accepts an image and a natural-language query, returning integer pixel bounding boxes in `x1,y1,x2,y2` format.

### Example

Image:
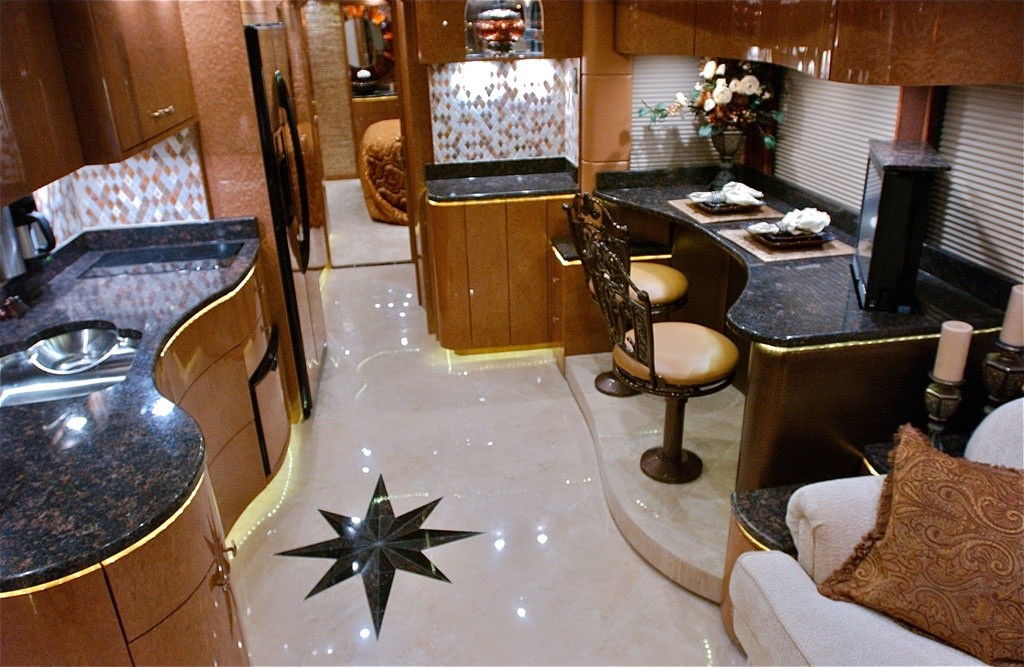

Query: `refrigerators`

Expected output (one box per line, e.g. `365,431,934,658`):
246,21,328,419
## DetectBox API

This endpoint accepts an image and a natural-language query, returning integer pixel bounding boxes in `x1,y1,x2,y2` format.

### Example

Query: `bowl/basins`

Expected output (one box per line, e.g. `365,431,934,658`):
352,80,379,95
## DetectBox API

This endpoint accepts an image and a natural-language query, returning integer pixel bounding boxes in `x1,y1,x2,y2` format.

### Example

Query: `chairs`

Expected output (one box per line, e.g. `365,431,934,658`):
562,192,738,485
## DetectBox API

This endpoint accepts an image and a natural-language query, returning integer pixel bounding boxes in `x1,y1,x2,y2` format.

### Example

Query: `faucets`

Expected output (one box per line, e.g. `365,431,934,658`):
0,295,29,323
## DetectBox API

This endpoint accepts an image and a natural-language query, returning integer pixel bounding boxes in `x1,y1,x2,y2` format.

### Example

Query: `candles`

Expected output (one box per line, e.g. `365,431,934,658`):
934,284,1024,381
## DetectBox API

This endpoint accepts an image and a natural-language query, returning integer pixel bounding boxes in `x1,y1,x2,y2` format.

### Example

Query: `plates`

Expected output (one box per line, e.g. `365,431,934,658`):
688,195,763,212
739,224,837,249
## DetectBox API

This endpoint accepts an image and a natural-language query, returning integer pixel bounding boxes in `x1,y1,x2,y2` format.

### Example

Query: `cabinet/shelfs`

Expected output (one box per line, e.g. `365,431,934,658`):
1,256,293,667
615,0,1024,86
0,0,199,206
553,254,671,355
721,518,772,656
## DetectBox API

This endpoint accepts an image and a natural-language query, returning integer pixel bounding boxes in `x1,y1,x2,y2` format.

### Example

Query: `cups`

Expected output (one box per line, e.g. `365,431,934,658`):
8,196,56,271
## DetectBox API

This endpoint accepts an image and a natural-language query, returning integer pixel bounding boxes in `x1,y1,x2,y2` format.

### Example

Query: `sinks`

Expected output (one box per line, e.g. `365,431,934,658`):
0,318,142,407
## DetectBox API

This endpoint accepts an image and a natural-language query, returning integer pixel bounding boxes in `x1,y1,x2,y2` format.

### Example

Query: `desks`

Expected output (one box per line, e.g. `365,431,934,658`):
593,184,1005,604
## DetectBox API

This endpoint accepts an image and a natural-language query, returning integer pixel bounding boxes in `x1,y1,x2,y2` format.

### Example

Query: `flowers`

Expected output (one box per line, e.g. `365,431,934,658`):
638,56,785,151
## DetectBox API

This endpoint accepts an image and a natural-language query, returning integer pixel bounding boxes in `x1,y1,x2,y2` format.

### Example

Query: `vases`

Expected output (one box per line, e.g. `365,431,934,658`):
712,130,745,190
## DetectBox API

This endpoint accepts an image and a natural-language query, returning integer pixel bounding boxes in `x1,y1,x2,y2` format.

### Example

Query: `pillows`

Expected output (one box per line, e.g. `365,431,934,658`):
816,423,1024,667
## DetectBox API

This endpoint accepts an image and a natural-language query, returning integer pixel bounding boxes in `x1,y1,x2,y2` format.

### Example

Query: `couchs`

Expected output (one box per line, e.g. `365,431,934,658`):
729,396,1023,666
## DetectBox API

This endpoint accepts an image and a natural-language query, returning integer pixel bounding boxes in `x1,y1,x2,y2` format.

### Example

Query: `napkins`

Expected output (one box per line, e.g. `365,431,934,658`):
685,181,831,236
